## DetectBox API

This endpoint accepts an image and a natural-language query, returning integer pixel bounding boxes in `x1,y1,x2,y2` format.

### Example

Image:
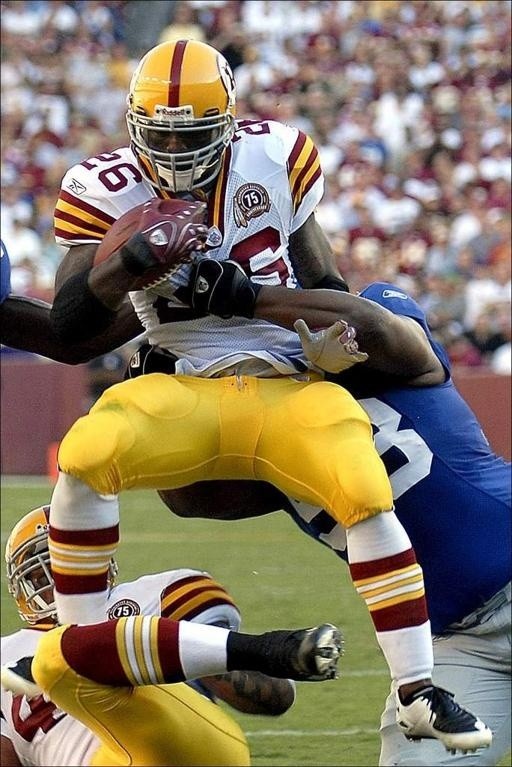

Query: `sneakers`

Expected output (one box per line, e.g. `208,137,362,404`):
2,656,43,701
267,622,344,681
396,684,493,755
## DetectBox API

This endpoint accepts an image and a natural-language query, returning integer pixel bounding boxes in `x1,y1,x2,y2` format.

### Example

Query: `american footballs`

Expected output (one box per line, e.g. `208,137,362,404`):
93,198,208,291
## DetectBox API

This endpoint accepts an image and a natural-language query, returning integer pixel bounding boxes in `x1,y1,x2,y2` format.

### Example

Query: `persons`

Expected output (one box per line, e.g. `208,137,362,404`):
2,503,348,765
46,34,494,757
158,253,510,766
0,2,512,373
0,235,144,362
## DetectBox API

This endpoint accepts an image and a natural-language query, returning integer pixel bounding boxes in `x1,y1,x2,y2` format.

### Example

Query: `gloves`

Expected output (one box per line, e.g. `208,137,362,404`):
122,344,171,380
123,196,208,281
177,259,262,320
293,318,369,374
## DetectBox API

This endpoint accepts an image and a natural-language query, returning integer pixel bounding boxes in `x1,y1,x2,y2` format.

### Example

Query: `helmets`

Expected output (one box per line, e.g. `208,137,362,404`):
125,39,238,194
5,505,119,625
